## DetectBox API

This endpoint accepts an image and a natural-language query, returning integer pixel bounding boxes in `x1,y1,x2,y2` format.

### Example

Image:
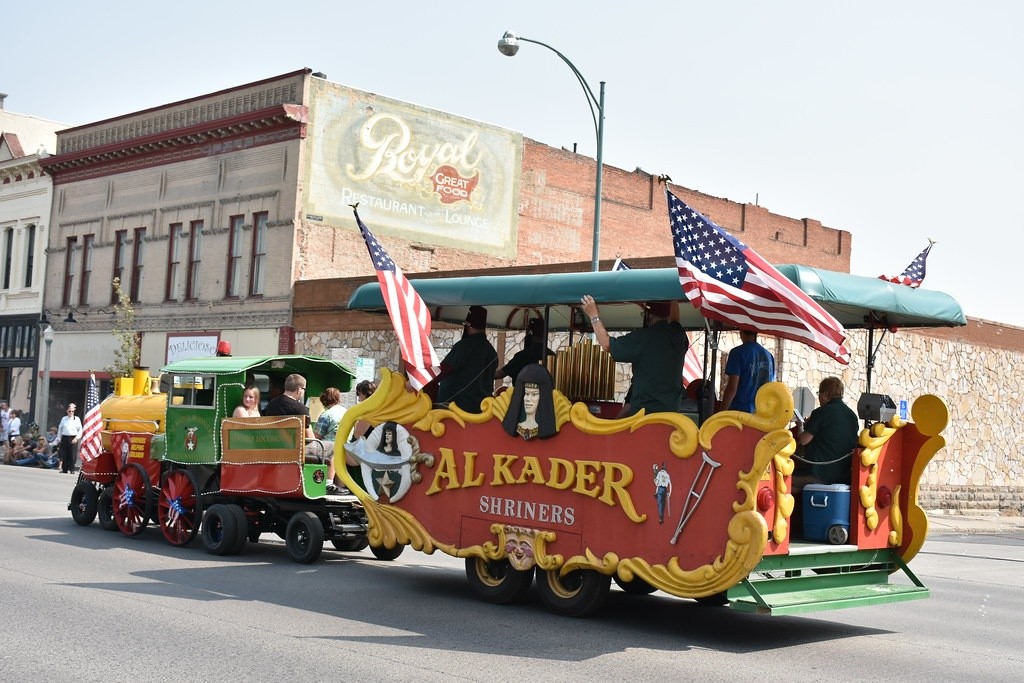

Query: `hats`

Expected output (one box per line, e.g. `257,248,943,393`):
465,305,488,329
526,317,545,343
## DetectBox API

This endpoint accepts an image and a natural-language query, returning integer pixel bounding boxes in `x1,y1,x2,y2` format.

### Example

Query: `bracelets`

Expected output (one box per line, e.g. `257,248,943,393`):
591,317,600,323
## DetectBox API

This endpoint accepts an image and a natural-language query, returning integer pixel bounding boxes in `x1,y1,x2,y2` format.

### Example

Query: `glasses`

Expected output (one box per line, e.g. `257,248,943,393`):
68,410,74,412
39,439,44,441
356,391,362,396
816,391,822,395
640,311,647,318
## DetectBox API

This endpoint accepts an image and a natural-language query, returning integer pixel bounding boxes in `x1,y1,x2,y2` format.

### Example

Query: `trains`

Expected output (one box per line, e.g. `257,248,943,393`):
67,344,406,565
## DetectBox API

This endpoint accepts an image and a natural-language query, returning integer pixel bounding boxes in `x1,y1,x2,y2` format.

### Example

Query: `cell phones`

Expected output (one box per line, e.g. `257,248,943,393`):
794,409,806,424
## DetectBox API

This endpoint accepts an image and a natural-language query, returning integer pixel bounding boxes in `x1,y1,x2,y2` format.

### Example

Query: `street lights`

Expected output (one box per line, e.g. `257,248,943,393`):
37,325,56,436
496,29,605,348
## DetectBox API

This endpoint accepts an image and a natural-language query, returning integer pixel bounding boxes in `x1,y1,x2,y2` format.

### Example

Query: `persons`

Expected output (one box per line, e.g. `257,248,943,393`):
0,400,21,447
581,295,689,418
262,374,350,494
790,376,859,496
232,385,260,417
0,426,63,469
313,387,354,442
344,380,379,466
494,318,556,387
58,403,82,474
718,329,776,414
406,306,498,416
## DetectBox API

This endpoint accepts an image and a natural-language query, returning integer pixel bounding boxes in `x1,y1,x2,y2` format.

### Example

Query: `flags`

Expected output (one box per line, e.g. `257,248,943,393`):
877,247,926,333
356,216,439,392
666,190,852,364
80,377,102,463
616,261,704,388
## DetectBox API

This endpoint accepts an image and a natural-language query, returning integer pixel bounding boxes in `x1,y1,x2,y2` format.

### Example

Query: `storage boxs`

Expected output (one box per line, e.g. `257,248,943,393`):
800,483,849,546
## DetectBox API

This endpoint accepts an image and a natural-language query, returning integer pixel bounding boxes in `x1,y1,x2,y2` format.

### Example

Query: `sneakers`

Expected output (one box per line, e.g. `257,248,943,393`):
326,483,350,495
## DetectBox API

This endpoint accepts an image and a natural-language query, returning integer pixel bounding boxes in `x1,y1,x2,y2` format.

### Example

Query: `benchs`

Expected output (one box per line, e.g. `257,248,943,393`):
312,422,368,495
219,416,328,501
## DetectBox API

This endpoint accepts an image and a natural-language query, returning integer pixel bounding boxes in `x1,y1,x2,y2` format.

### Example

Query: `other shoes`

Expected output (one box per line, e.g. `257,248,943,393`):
60,470,68,473
10,456,16,464
71,470,75,474
